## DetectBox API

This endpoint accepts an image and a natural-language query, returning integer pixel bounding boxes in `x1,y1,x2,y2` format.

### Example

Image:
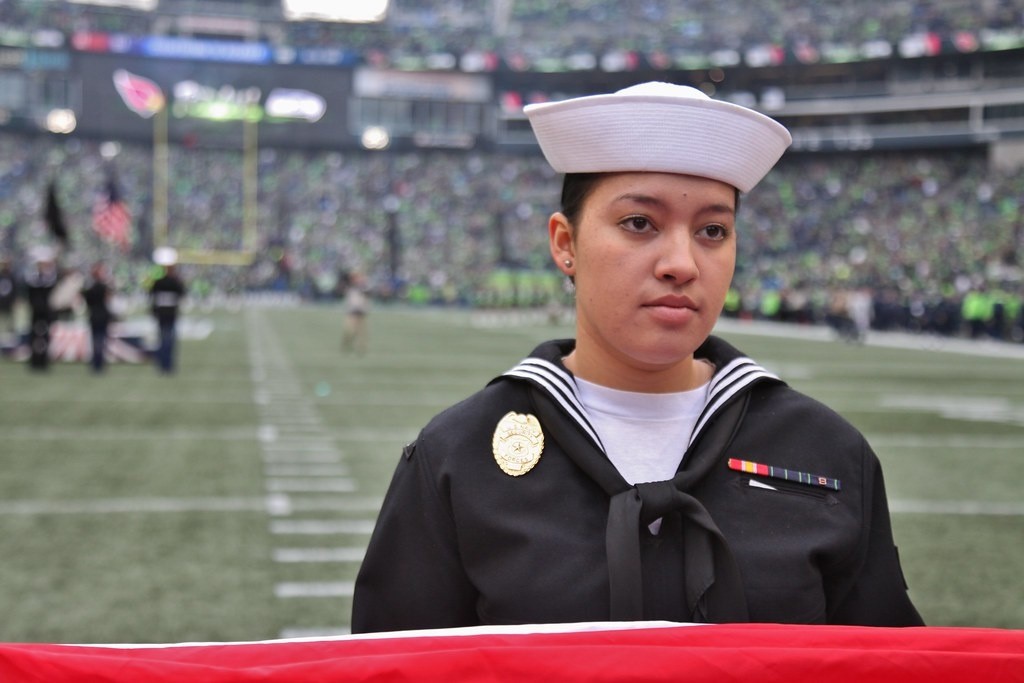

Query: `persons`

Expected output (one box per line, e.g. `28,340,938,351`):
0,0,1024,379
350,80,928,635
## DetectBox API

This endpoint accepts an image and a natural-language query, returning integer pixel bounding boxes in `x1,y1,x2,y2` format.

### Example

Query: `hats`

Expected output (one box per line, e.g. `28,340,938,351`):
522,82,793,194
